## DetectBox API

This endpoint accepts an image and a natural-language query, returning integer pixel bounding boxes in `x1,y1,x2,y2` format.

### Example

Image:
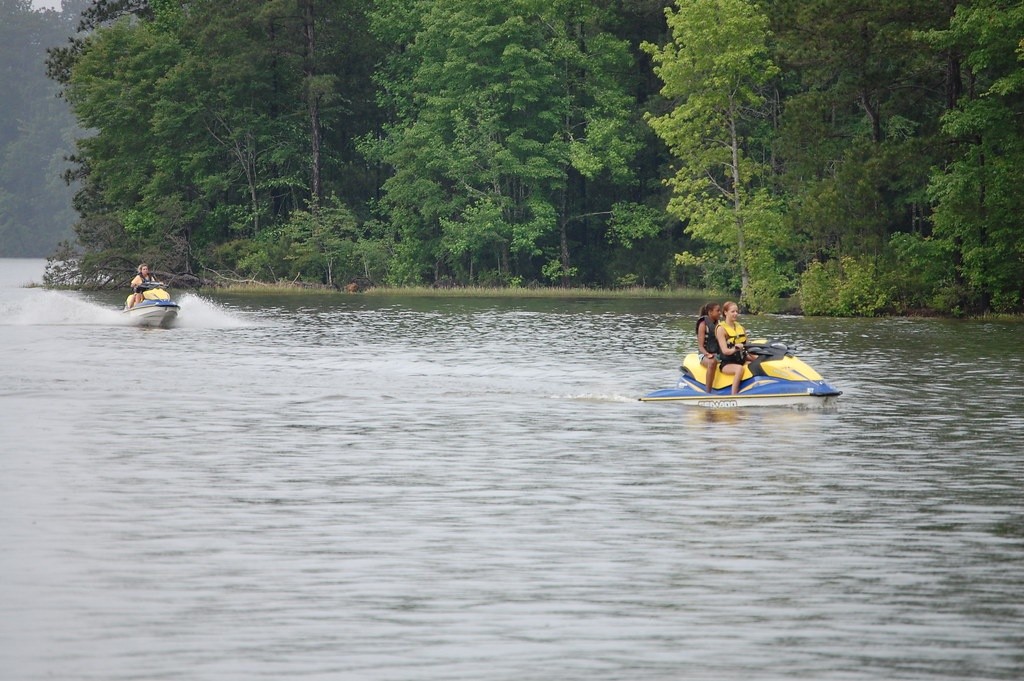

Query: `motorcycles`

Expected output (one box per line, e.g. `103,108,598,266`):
121,281,181,328
638,339,843,407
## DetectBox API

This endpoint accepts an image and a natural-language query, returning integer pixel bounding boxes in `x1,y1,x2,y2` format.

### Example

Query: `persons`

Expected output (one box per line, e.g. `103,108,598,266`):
714,302,759,396
695,302,721,396
125,264,164,311
